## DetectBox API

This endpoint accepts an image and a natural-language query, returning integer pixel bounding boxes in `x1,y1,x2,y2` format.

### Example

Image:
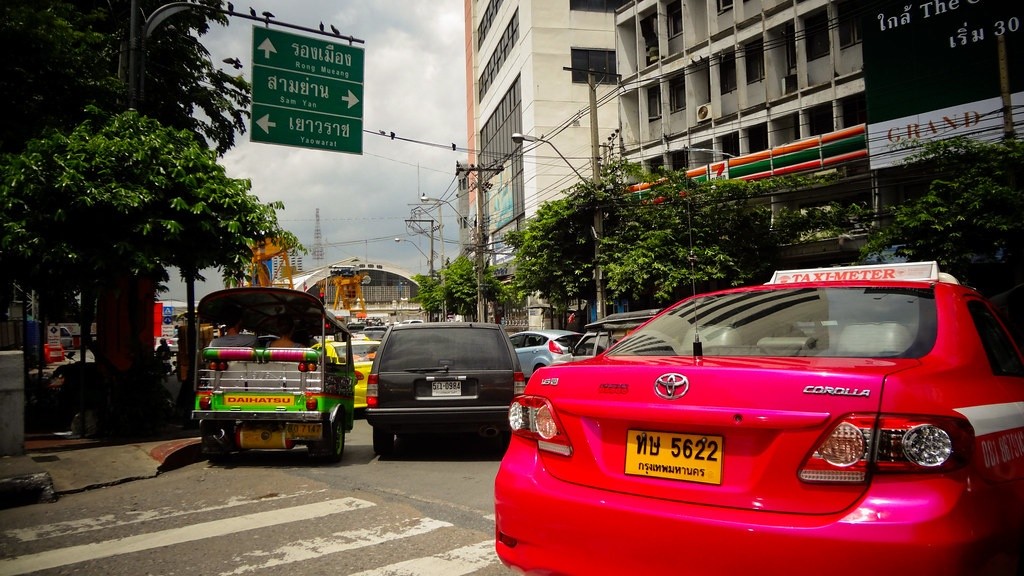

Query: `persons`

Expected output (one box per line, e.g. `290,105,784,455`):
157,338,174,376
207,308,305,347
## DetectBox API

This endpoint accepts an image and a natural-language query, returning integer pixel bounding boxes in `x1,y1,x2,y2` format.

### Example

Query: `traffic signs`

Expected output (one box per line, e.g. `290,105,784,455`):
249,27,365,158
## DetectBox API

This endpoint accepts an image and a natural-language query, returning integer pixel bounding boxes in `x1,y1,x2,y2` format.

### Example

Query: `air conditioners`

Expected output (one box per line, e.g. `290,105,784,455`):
696,102,712,122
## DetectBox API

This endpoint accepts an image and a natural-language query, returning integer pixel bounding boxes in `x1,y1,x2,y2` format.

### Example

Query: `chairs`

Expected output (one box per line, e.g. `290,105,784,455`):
679,325,742,355
836,321,912,356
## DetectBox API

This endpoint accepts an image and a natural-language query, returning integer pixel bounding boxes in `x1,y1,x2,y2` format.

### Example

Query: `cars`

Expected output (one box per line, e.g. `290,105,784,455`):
508,330,588,380
574,331,627,361
312,320,424,408
366,322,529,461
59,328,73,349
169,335,179,354
495,262,1024,575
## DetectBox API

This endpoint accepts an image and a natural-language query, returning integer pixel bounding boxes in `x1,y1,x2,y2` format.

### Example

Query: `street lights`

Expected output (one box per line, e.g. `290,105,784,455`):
420,197,484,323
395,239,434,322
510,132,603,321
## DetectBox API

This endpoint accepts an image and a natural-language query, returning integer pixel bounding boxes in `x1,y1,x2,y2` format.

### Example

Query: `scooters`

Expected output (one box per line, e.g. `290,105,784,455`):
192,287,357,467
